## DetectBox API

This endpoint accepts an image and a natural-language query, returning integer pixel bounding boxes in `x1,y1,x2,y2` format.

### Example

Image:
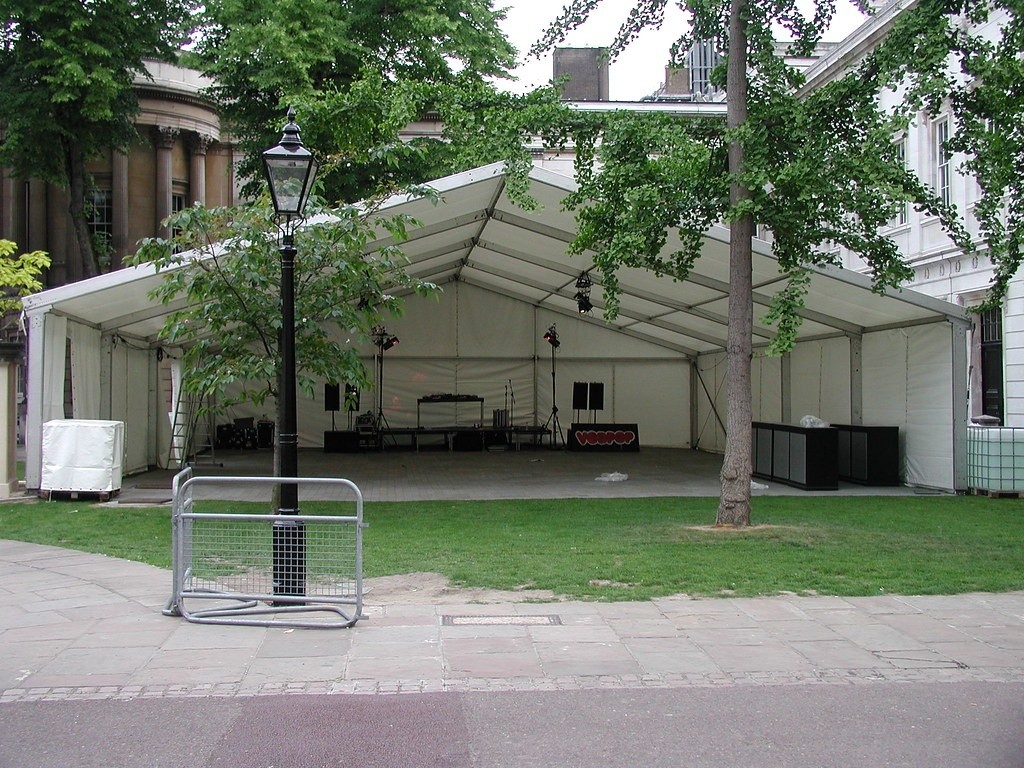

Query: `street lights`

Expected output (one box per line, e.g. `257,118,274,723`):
261,103,321,611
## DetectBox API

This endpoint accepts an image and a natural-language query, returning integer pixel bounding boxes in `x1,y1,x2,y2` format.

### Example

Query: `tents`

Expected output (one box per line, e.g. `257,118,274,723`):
24,155,976,494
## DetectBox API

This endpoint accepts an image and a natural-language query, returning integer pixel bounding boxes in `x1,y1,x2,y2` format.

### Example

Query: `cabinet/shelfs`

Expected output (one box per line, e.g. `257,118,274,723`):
831,421,900,487
752,421,839,491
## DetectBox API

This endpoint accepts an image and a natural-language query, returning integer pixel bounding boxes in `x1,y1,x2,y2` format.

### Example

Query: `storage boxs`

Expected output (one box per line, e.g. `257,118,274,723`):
233,416,255,430
256,421,274,450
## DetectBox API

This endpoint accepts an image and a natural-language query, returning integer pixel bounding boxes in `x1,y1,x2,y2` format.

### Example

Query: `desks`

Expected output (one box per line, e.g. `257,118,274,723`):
40,418,126,503
416,398,484,428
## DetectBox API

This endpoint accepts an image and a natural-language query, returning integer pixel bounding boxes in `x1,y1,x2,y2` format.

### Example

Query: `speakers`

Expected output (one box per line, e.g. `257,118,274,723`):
345,383,360,411
325,383,340,412
589,382,604,410
573,382,588,409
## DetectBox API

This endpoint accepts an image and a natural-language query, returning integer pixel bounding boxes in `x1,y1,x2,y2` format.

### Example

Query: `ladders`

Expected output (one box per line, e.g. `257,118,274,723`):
165,377,216,471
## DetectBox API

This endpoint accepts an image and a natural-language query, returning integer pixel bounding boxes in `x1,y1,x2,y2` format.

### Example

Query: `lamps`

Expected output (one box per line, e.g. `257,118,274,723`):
579,303,586,313
573,292,593,312
543,332,561,348
383,337,400,351
